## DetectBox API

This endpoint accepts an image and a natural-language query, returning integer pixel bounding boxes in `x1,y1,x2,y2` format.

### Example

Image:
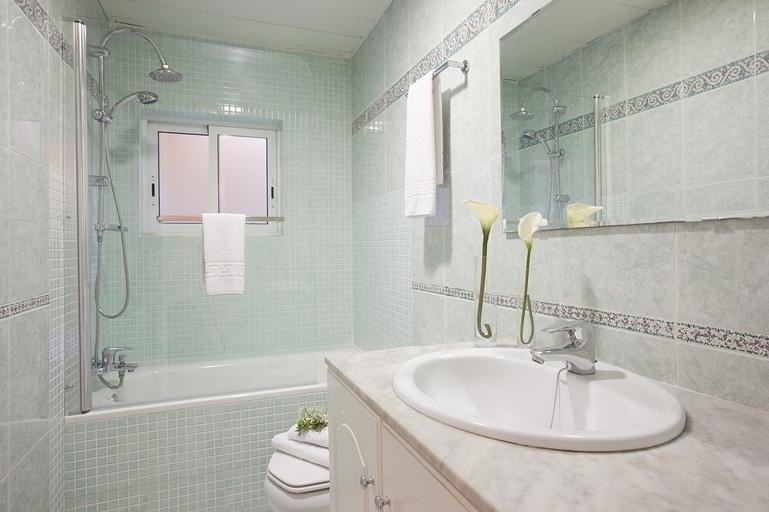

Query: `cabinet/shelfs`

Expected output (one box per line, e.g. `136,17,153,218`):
327,370,481,512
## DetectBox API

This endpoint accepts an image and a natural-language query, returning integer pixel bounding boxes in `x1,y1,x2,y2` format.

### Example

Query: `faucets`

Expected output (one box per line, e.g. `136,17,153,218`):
109,361,140,372
530,319,594,377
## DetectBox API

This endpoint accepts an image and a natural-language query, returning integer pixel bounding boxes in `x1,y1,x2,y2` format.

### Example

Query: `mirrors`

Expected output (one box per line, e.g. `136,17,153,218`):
499,0,769,231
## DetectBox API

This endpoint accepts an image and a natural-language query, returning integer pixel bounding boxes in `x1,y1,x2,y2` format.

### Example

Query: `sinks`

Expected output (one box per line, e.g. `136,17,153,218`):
394,349,688,452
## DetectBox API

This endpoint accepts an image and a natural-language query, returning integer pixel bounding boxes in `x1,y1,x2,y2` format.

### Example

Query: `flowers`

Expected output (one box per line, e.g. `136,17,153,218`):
566,204,603,227
464,199,499,338
295,406,328,432
519,212,548,344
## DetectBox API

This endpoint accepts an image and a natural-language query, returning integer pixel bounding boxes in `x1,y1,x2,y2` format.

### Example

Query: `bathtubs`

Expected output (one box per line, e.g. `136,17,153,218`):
84,346,368,414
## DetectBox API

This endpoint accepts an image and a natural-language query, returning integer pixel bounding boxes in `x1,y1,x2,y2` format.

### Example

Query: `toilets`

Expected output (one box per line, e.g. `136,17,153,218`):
263,446,330,511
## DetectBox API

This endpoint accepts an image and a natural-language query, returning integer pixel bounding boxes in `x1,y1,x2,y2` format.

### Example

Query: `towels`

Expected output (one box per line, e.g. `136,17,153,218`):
404,70,443,218
271,423,330,467
202,212,246,295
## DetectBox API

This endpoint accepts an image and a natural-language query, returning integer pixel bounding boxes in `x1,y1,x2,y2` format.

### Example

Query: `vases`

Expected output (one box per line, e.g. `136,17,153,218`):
474,255,496,346
517,256,536,346
